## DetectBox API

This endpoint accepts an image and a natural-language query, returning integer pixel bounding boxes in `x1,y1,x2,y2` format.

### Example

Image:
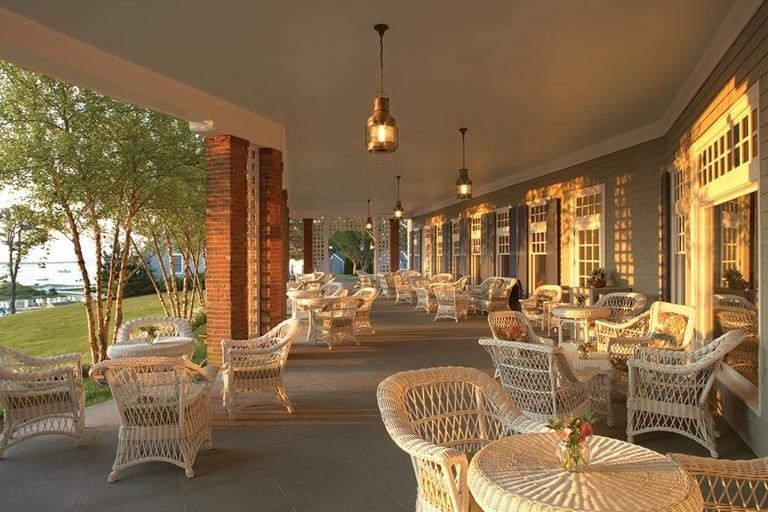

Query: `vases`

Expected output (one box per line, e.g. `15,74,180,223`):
592,282,607,288
145,332,155,345
557,434,590,473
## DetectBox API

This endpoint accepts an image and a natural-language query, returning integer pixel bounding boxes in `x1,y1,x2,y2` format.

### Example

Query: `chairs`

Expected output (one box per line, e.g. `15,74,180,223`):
477,333,602,431
1,341,87,456
219,316,301,423
593,308,651,352
661,450,768,512
286,269,379,351
714,285,760,386
486,310,555,381
619,328,751,459
379,270,519,324
114,314,196,362
372,362,557,512
584,292,646,351
519,283,562,332
88,357,220,482
606,298,698,419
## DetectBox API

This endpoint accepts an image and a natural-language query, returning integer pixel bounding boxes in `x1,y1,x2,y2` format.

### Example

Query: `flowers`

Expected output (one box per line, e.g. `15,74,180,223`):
141,324,158,333
550,411,596,444
587,270,609,282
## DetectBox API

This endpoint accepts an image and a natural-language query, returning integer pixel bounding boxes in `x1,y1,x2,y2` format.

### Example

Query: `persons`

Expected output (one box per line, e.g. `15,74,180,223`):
0,303,6,317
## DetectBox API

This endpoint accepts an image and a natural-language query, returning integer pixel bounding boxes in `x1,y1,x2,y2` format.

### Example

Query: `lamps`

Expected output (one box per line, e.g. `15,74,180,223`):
455,128,473,200
393,176,404,222
367,23,399,154
365,199,373,230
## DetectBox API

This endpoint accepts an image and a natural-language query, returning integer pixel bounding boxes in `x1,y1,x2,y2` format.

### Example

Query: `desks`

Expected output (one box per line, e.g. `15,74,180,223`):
553,306,612,345
466,431,705,510
569,285,632,341
106,335,196,362
